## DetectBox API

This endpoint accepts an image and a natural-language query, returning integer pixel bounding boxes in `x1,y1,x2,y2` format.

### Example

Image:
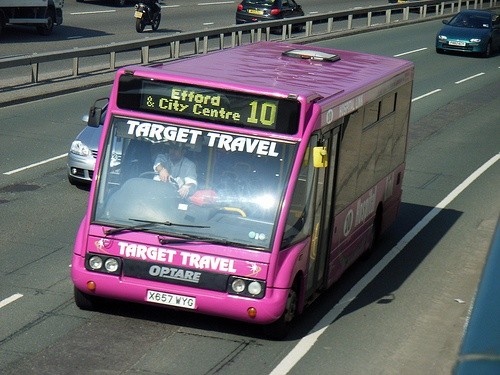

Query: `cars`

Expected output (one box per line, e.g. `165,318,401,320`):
435,9,499,56
236,0,306,32
64,100,108,182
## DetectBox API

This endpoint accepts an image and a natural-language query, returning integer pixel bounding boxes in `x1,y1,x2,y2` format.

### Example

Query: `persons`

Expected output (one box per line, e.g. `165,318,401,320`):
460,15,471,26
143,0,161,24
152,140,198,200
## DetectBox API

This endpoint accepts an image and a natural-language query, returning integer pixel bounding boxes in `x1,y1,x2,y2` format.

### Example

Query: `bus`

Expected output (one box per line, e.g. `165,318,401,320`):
70,40,414,336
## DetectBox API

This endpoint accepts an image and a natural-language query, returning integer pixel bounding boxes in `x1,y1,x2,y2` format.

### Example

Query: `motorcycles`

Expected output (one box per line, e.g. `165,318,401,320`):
133,0,164,32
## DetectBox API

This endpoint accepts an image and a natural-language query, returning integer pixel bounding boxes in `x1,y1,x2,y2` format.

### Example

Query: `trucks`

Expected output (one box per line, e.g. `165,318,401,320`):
0,0,64,33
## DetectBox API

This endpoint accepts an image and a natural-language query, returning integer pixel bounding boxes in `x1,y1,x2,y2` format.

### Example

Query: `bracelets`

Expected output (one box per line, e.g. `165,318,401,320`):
158,167,164,173
